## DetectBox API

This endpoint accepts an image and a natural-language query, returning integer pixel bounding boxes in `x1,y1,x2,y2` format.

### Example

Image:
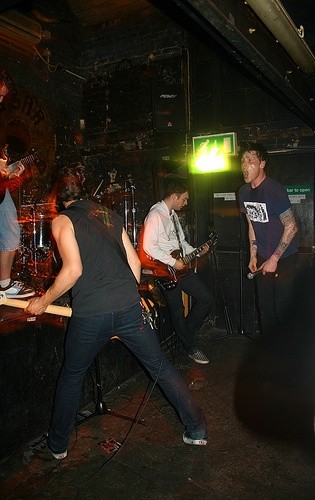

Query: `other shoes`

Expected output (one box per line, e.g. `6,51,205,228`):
46,432,68,459
188,349,209,364
182,430,207,445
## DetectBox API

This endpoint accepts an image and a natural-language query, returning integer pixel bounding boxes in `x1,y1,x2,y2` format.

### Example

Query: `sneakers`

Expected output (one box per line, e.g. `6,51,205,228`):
0,280,36,298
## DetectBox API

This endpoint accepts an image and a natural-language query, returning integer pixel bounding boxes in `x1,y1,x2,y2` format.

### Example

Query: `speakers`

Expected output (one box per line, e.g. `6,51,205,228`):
287,193,315,252
267,148,315,193
274,253,315,333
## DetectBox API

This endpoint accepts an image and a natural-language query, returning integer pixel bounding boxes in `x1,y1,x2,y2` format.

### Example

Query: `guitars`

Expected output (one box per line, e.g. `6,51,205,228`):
0,146,42,183
149,232,220,291
0,291,73,318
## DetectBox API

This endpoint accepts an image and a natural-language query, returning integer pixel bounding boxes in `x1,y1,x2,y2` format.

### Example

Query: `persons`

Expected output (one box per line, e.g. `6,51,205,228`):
24,174,209,459
0,158,35,298
238,145,298,341
142,179,212,364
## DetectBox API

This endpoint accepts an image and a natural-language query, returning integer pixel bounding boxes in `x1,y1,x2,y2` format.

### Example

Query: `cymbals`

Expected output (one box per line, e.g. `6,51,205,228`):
14,202,59,223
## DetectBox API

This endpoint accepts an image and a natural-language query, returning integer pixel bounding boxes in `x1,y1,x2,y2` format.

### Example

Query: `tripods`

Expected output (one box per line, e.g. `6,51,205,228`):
42,350,148,437
209,213,262,349
